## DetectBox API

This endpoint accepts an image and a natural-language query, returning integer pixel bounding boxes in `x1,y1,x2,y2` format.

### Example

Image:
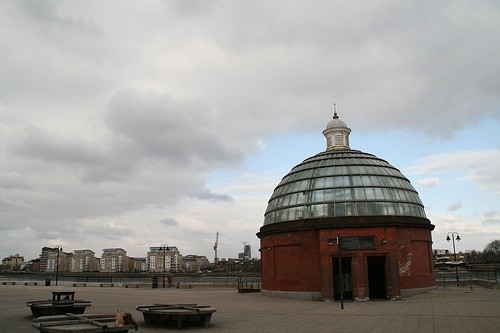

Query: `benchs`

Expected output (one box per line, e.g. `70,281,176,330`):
73,283,86,287
3,281,16,285
24,282,37,285
177,282,191,288
125,283,139,288
100,283,114,287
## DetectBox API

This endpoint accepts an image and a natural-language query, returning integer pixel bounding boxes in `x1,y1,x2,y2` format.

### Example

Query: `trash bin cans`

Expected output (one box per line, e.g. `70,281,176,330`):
152,279,158,289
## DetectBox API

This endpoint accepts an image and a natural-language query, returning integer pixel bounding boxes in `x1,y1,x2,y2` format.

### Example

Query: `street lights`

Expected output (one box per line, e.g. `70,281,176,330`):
53,245,64,285
445,231,462,282
160,243,171,288
